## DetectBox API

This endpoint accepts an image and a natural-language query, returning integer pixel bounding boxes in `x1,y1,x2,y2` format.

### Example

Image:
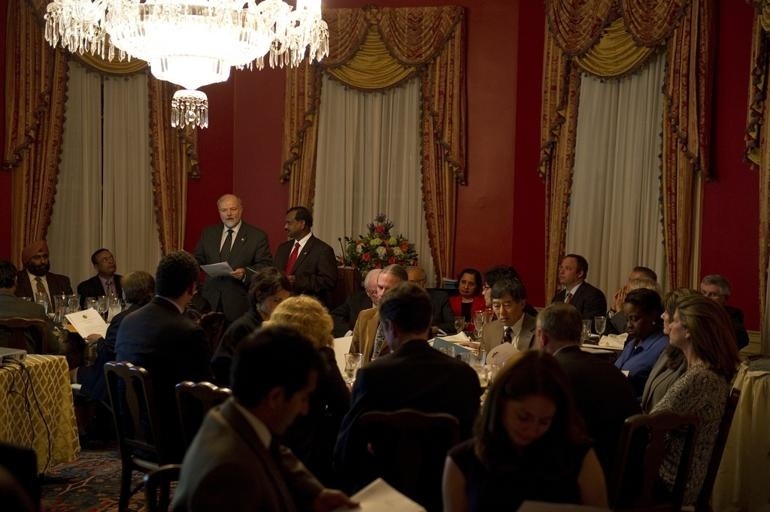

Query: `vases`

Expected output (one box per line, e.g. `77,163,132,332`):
360,270,368,289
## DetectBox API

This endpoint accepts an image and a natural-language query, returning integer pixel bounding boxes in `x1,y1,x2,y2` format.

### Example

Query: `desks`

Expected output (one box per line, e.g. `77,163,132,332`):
2,355,82,465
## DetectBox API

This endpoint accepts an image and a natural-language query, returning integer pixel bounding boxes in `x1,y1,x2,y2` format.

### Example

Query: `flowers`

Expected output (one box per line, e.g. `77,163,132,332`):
346,213,419,272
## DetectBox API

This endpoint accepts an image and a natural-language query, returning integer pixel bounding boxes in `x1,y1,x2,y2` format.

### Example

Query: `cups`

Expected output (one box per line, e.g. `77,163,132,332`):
344,352,362,381
54,294,66,324
68,294,80,313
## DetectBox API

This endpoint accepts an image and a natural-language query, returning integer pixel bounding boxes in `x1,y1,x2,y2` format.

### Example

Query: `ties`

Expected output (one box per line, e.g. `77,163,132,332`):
565,293,572,306
286,242,300,274
219,229,234,262
33,277,52,314
106,280,114,299
504,327,512,344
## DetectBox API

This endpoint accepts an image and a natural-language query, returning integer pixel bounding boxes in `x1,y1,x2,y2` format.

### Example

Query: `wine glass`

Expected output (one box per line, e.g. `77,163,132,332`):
593,315,609,341
453,315,466,334
98,294,110,322
472,308,489,339
486,306,494,326
583,321,593,348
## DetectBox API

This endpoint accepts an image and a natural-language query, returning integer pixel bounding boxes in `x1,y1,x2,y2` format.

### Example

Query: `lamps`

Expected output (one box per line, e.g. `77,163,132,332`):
44,1,329,130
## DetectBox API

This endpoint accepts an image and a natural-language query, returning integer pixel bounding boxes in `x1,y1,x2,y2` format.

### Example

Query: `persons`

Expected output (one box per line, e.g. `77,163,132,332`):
537,304,642,483
0,259,69,358
14,241,73,316
78,249,126,323
405,265,429,291
624,276,668,301
607,267,657,335
443,351,610,512
171,326,361,512
641,288,703,411
449,267,490,331
551,253,607,334
274,206,345,312
345,265,407,378
194,193,270,325
613,289,671,397
333,283,482,498
702,276,750,351
261,294,352,490
478,278,542,362
363,270,383,307
68,271,153,399
206,268,292,391
115,252,215,466
649,296,741,511
484,265,537,316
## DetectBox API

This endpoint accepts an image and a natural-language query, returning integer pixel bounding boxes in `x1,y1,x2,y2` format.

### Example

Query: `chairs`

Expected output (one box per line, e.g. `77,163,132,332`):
662,387,742,512
175,381,232,461
0,317,52,354
357,412,460,462
621,409,680,454
104,362,174,511
